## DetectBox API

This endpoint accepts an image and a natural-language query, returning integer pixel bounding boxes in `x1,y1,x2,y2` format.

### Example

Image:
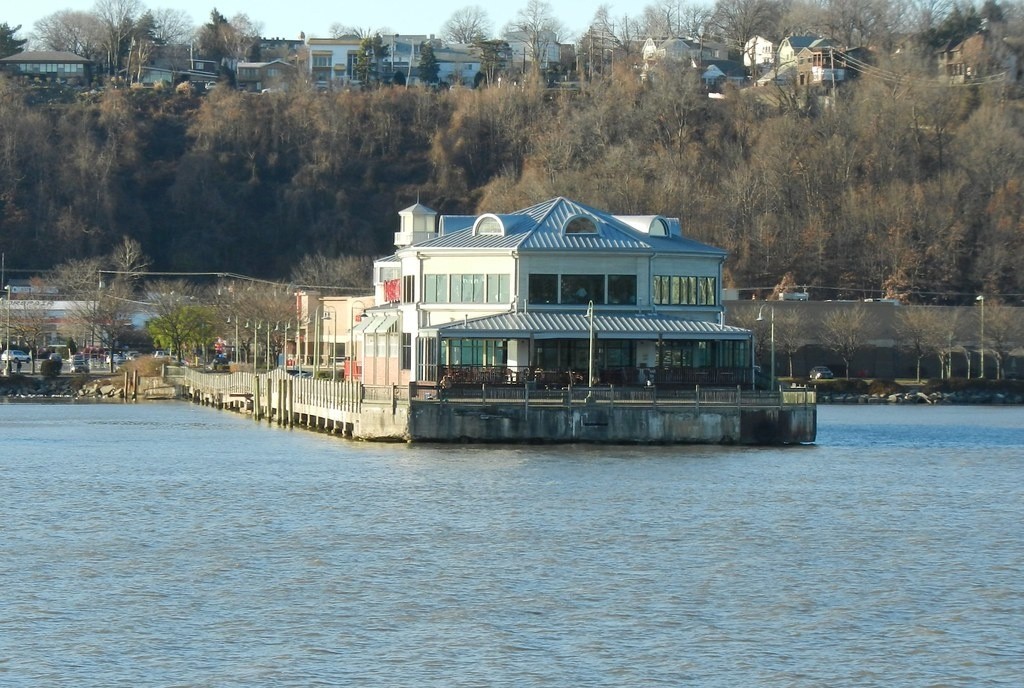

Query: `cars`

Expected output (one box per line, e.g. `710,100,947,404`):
808,366,834,379
49,350,229,373
1,350,32,364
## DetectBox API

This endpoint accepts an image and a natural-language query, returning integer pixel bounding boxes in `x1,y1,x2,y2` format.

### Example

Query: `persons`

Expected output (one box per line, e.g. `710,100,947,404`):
17,360,22,375
440,375,450,401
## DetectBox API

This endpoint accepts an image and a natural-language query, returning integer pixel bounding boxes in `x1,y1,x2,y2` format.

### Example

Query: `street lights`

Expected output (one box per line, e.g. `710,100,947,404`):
305,310,321,373
313,304,327,380
225,311,239,365
975,296,988,379
273,319,289,380
3,284,13,378
324,306,338,383
285,316,303,378
258,318,270,374
243,316,257,375
756,302,775,391
350,299,369,383
584,299,597,404
